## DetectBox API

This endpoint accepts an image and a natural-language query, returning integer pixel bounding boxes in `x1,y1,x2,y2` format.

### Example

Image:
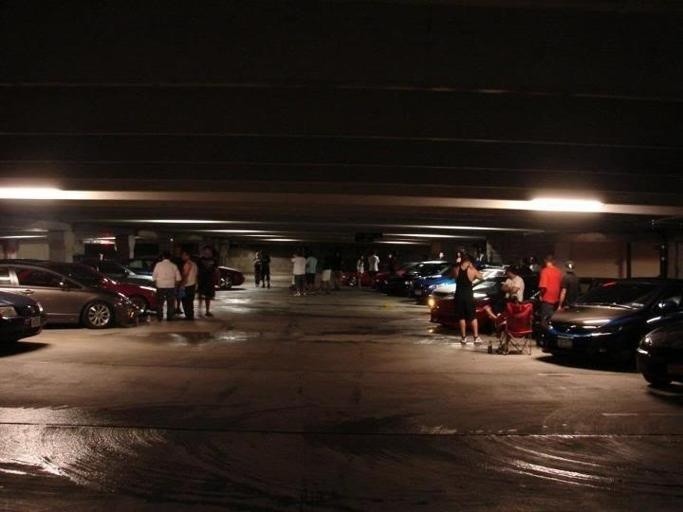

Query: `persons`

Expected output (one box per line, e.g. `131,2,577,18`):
435,252,446,261
304,250,318,295
475,250,484,267
178,250,198,321
171,246,186,314
289,250,306,297
386,253,396,275
450,254,484,344
537,252,563,349
455,251,462,263
150,250,182,321
481,294,520,355
251,251,264,288
527,255,540,271
195,244,217,319
258,247,270,289
499,265,525,304
319,255,331,294
554,259,579,310
356,255,365,289
365,247,380,289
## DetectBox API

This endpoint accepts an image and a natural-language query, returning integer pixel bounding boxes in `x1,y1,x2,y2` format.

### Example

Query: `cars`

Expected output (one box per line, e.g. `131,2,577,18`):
539,275,683,366
633,304,683,388
0,286,49,353
337,258,612,337
0,251,248,330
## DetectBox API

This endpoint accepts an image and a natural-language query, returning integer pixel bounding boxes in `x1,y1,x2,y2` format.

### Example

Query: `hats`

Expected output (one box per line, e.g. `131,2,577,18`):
565,260,576,273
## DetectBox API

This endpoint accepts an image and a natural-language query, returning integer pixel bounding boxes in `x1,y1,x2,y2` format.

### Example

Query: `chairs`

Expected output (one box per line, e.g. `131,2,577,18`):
497,302,533,357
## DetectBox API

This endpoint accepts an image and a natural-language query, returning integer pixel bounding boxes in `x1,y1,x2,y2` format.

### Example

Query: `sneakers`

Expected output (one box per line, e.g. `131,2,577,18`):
459,336,466,343
292,289,308,297
473,337,482,343
156,307,214,322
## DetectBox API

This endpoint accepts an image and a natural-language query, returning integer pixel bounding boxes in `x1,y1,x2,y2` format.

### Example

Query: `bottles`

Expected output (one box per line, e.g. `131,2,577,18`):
486,339,492,353
